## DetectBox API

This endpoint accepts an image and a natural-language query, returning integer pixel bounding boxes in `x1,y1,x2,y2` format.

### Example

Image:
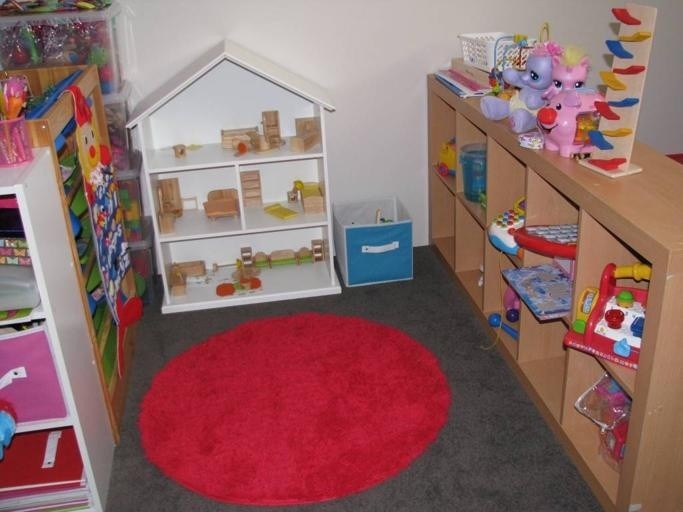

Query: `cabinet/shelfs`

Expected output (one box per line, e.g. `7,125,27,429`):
428,71,683,512
1,64,140,443
126,39,342,314
1,145,115,510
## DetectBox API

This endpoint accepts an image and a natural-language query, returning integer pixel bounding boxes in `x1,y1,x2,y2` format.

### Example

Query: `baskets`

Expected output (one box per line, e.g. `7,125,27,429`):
458,32,538,74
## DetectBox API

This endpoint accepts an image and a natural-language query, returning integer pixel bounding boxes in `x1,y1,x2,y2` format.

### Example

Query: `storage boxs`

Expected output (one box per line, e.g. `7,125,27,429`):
333,198,415,288
0,0,156,305
